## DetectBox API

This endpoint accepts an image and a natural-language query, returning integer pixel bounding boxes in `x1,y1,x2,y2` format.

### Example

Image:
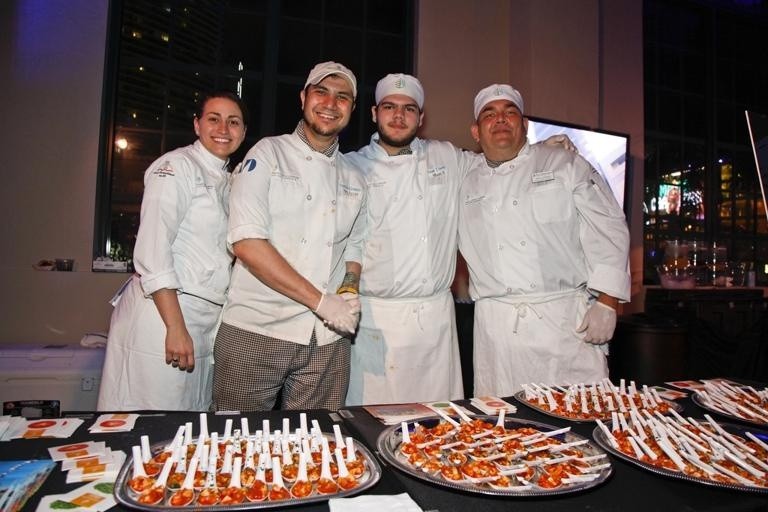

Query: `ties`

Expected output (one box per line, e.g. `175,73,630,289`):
656,182,683,218
523,114,630,222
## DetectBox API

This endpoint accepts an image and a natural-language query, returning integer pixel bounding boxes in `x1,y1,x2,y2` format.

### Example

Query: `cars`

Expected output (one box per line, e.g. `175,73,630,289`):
55,257,73,271
658,239,697,288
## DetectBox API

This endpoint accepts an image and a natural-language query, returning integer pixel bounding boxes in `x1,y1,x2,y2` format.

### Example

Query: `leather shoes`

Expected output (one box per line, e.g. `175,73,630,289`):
315,290,361,336
577,303,617,345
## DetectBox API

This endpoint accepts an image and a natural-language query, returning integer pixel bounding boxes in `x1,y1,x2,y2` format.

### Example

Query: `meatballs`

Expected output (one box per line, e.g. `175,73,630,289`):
613,422,766,489
715,389,766,423
401,418,583,488
128,440,364,507
528,386,673,421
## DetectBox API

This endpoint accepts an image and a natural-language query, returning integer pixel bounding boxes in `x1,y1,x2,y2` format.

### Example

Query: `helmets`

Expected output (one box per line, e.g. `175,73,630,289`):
32,258,54,270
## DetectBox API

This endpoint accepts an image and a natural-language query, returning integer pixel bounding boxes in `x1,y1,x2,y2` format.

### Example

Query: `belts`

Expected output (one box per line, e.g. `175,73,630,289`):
704,242,727,285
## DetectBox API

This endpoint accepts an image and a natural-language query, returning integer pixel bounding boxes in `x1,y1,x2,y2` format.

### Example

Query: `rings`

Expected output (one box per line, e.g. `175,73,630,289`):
173,358,179,362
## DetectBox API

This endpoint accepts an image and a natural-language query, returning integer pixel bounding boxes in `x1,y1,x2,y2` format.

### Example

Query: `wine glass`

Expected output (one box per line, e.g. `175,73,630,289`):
704,242,727,285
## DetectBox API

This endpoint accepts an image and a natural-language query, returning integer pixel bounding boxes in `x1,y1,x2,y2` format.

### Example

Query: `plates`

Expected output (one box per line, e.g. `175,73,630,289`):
32,258,54,270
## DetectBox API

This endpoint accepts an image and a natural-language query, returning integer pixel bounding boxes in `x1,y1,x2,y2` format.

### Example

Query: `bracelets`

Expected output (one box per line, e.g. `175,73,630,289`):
338,286,358,294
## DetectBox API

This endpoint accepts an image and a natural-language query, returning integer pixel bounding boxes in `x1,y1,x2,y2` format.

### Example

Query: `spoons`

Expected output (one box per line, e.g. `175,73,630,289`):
596,409,767,488
131,412,364,505
518,378,768,424
401,404,611,491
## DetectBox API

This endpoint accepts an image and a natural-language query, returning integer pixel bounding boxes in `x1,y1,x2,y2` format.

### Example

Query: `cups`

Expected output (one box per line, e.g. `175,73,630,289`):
658,239,697,288
55,257,73,271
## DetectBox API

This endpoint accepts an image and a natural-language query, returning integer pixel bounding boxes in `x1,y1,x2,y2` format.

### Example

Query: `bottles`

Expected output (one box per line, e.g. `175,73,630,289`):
375,73,424,111
474,83,525,120
304,61,358,101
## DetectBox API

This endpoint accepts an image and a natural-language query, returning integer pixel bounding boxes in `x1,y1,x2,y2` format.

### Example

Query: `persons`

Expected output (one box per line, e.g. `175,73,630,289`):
211,62,368,410
228,74,578,406
98,89,248,411
453,84,631,398
